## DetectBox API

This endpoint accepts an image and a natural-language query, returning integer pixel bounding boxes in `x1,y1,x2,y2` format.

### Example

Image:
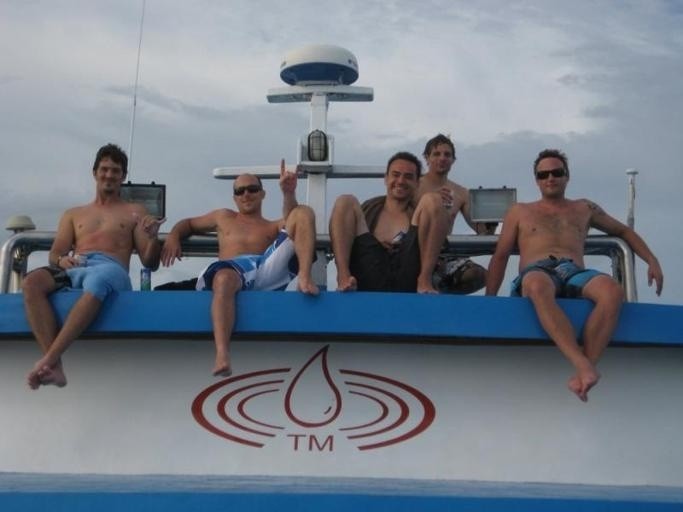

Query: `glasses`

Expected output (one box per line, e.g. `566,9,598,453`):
234,184,260,195
535,169,567,179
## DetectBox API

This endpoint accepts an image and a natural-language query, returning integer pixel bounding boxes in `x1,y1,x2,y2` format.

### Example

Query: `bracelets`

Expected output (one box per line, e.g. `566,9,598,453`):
56,255,64,266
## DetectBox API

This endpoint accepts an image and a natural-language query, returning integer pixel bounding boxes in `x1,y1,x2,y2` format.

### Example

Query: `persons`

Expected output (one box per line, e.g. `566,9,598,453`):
161,157,319,375
411,133,497,295
328,152,446,295
486,149,662,402
22,143,167,389
